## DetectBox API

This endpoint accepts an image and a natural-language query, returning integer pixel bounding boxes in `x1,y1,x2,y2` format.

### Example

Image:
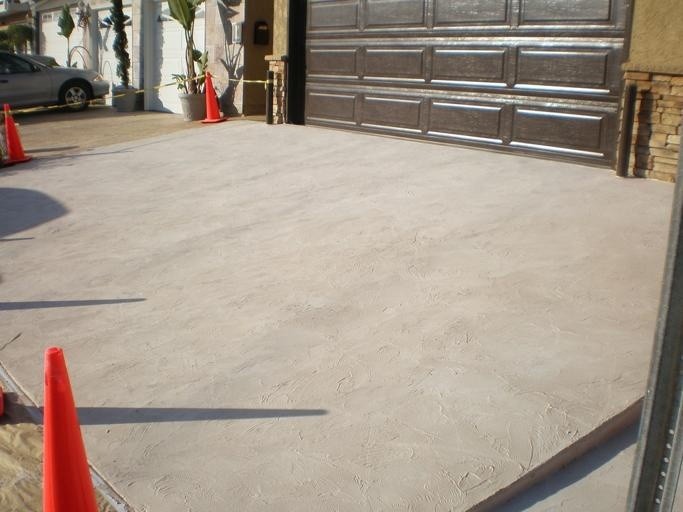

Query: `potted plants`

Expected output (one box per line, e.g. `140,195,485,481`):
109,0,137,113
166,0,209,121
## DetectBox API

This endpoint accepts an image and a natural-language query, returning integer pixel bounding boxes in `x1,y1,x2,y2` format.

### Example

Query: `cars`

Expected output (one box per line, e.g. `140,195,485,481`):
0,51,109,110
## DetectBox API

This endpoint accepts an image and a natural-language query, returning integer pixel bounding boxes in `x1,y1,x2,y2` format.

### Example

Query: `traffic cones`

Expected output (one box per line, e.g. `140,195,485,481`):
202,72,226,123
42,348,98,511
1,102,32,166
